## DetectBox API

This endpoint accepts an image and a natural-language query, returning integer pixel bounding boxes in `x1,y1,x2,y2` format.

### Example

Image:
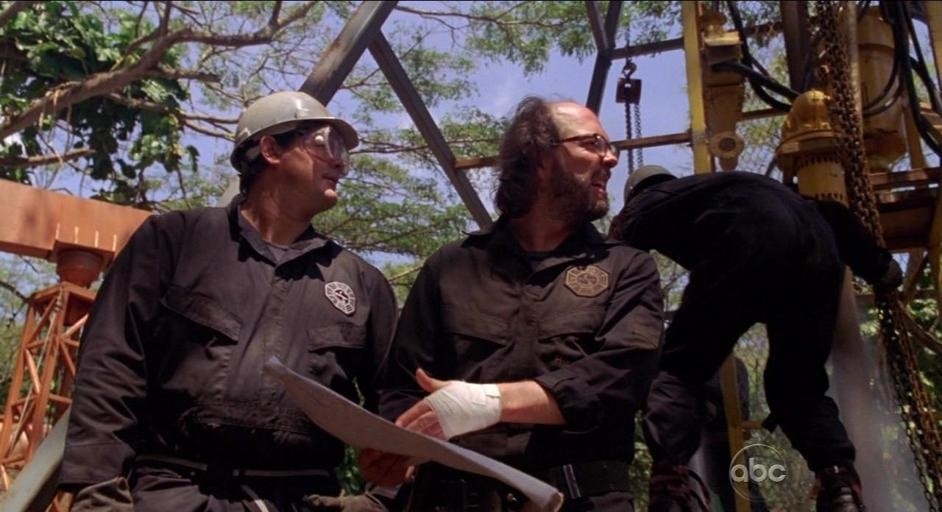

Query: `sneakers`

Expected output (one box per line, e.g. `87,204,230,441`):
817,479,862,512
649,492,703,512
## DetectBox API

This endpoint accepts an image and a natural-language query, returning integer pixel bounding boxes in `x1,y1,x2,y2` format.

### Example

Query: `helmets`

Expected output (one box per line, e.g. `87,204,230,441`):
230,91,358,173
624,164,676,202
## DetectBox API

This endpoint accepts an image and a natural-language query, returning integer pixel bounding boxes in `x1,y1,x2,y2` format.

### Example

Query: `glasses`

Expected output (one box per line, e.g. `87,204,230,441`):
300,126,349,176
556,131,621,161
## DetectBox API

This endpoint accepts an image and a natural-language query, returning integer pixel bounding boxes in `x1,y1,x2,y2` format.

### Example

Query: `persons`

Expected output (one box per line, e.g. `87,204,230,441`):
338,99,667,511
50,91,402,512
605,163,903,512
